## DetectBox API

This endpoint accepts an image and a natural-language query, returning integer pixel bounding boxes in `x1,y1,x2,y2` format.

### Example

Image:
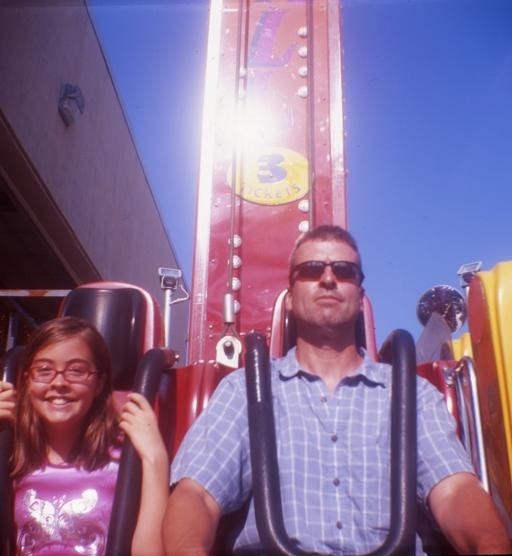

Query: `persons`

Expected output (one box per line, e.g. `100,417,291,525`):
162,224,512,556
0,314,169,556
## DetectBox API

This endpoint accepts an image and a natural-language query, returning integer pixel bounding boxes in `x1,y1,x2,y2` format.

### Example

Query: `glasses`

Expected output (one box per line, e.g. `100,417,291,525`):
24,361,100,385
290,259,365,282
